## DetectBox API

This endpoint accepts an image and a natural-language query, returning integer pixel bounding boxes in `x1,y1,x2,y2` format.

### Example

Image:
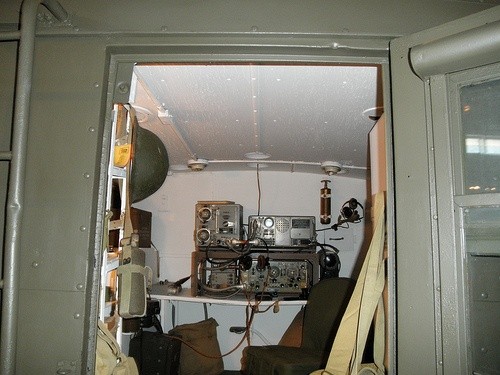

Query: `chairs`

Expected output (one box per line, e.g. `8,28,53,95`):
240,276,356,375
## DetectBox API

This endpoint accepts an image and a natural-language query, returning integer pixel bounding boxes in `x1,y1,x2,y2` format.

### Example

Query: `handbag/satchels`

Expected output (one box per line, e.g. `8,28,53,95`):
128,314,182,375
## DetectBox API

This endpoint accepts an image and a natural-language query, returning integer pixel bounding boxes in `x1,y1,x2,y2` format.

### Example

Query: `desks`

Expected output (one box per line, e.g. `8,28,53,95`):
145,288,308,370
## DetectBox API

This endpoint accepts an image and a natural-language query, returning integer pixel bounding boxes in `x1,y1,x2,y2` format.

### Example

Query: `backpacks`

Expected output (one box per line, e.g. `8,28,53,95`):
95,318,139,375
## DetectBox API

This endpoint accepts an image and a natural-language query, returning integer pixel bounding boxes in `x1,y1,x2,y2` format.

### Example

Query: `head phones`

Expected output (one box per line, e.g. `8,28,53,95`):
341,200,365,222
